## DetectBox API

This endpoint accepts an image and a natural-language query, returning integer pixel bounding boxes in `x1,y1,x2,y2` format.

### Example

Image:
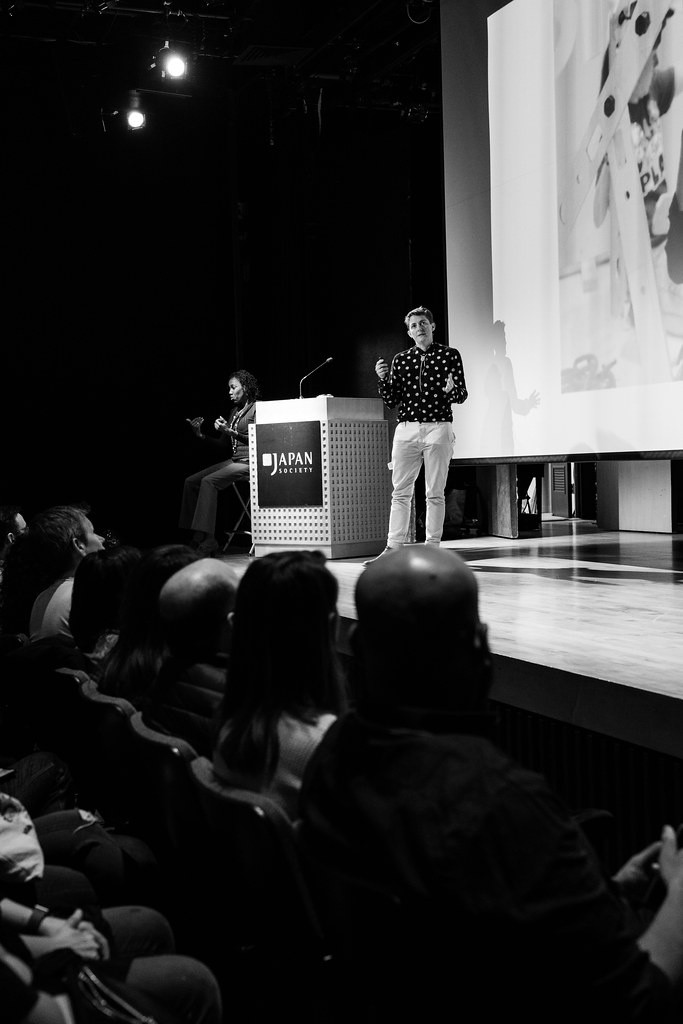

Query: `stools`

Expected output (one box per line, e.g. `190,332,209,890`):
223,481,257,557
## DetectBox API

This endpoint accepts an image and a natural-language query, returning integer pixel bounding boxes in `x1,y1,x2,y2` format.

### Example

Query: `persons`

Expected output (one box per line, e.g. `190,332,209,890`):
177,369,261,558
294,544,683,1024
0,505,243,758
0,751,223,1024
592,49,683,286
363,305,468,565
212,551,345,818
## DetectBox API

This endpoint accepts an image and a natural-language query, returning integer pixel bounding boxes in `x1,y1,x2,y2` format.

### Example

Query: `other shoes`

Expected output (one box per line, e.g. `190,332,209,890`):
362,544,393,566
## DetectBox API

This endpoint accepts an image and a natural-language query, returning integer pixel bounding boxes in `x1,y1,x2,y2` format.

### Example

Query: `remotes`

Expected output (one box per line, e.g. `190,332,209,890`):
378,357,389,378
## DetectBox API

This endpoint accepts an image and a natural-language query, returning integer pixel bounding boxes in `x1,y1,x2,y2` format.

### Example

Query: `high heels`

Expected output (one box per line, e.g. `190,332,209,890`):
195,539,219,558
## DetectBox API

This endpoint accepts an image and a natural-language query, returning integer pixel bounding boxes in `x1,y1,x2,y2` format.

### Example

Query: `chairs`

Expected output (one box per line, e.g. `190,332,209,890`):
0,619,335,973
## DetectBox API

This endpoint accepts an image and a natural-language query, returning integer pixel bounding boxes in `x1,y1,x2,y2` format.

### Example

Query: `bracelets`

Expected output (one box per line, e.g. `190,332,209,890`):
26,904,49,935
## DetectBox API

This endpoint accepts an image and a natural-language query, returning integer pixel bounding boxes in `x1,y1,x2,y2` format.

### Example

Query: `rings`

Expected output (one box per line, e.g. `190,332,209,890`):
197,422,199,424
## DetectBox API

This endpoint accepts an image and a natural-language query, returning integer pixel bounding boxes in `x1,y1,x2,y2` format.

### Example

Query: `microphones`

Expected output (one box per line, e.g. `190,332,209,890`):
299,357,333,399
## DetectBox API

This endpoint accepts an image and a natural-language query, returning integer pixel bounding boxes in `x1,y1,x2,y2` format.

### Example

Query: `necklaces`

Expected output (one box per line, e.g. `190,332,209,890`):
228,401,248,453
419,353,427,362
